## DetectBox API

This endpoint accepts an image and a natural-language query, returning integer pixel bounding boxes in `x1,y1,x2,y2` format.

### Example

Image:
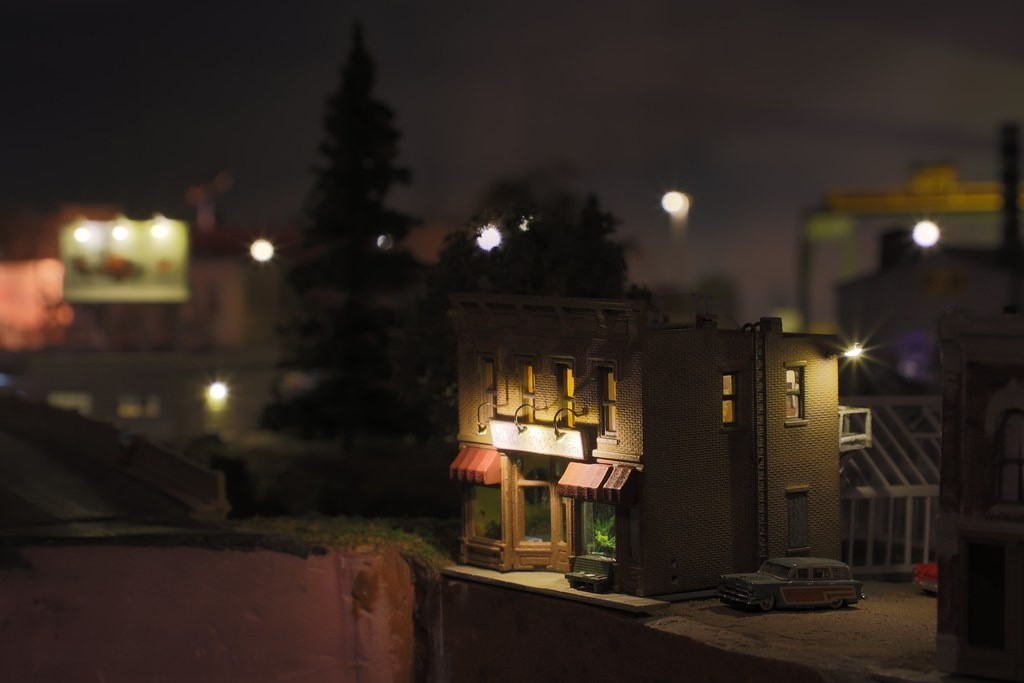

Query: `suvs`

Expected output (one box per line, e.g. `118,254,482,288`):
719,556,862,614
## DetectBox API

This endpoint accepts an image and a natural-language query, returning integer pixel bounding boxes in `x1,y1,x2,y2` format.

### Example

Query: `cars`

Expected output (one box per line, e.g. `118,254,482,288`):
911,561,939,597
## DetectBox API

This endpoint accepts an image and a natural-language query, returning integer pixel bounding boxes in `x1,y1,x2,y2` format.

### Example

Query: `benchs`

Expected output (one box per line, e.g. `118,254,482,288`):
564,556,614,593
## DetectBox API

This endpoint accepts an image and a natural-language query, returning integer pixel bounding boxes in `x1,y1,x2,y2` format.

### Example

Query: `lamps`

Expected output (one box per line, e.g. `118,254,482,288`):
476,402,507,434
514,404,549,436
552,407,588,442
